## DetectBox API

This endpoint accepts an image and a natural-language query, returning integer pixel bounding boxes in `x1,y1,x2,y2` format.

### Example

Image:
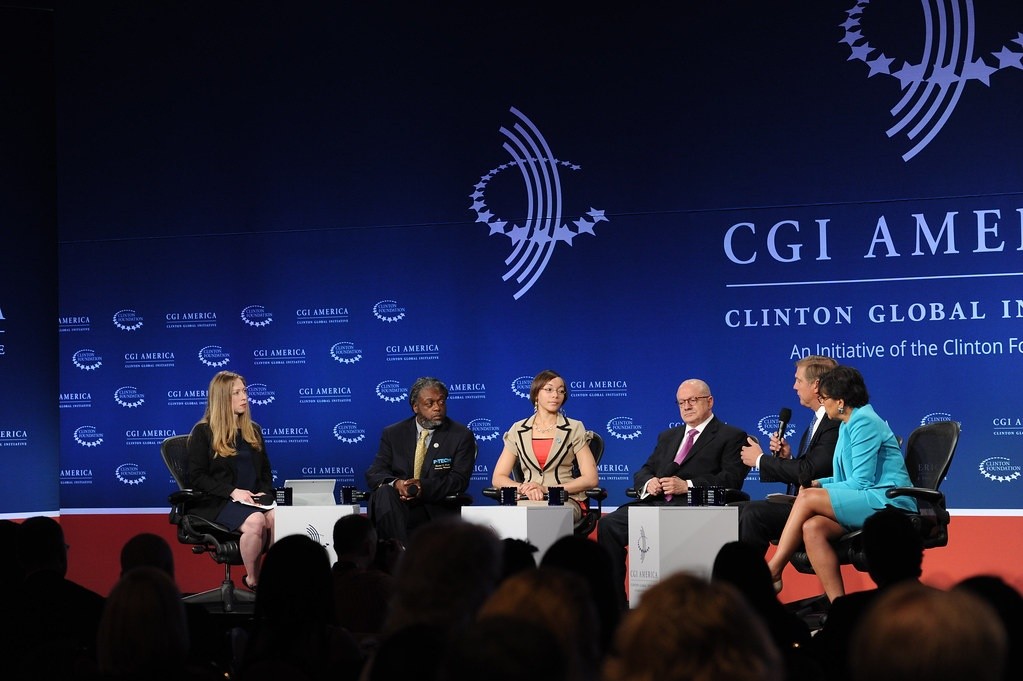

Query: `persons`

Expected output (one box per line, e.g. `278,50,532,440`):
366,378,476,545
0,507,1023,681
492,370,599,520
768,367,918,604
741,356,841,555
187,372,275,591
596,379,748,602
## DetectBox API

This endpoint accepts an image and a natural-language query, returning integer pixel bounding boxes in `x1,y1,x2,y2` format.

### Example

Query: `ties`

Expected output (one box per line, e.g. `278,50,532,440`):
414,430,430,479
788,414,819,497
664,428,699,502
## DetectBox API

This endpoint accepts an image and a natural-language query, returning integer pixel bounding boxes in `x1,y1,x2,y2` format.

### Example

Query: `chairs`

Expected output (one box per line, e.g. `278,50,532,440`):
768,421,960,628
484,431,606,539
161,434,257,611
357,436,479,504
625,436,760,504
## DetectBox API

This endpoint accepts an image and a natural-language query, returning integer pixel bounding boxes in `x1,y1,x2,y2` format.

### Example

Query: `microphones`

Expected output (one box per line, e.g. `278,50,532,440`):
772,408,793,458
251,495,273,506
519,493,549,500
406,484,418,496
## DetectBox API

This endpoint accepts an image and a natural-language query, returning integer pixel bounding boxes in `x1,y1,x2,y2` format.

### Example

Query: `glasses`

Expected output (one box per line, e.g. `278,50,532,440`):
677,396,711,406
817,396,832,403
539,386,566,396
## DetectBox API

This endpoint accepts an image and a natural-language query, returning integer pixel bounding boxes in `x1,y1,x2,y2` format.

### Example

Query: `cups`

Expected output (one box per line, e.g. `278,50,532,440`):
548,487,564,506
687,486,705,506
340,486,357,504
500,487,517,506
275,487,292,506
707,485,725,506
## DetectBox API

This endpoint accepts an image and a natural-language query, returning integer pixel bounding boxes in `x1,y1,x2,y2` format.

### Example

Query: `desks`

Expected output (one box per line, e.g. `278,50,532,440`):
273,504,361,568
461,504,574,568
627,504,739,611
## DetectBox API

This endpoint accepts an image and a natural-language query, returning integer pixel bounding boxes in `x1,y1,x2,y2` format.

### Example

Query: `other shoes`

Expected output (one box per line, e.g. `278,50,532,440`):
241,574,258,591
773,579,785,596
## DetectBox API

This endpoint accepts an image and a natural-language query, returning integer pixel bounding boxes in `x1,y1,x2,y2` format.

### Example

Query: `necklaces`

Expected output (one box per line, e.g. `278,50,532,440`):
535,422,557,433
